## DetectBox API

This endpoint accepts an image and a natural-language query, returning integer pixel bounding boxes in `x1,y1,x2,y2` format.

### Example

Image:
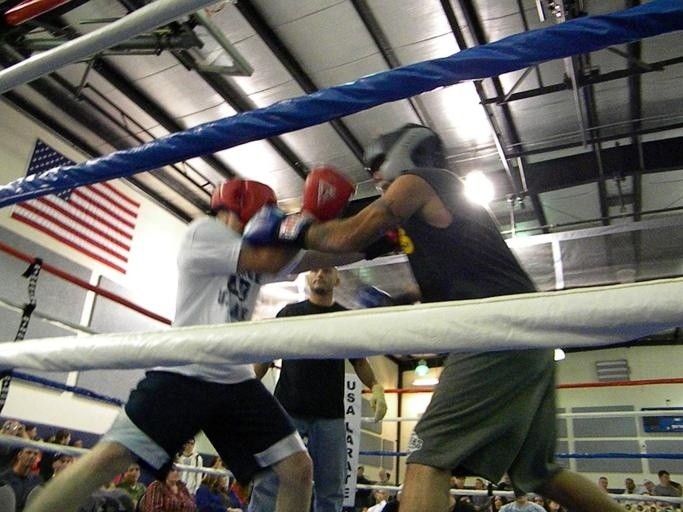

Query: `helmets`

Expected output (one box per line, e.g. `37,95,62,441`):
210,179,277,230
363,123,438,180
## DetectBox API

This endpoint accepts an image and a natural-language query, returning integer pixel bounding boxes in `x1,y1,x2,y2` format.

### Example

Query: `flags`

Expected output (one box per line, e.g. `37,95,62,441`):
9,137,141,275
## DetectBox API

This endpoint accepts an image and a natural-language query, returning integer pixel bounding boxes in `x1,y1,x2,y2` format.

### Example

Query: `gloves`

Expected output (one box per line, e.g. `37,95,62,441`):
359,230,401,260
243,167,356,250
370,384,387,423
